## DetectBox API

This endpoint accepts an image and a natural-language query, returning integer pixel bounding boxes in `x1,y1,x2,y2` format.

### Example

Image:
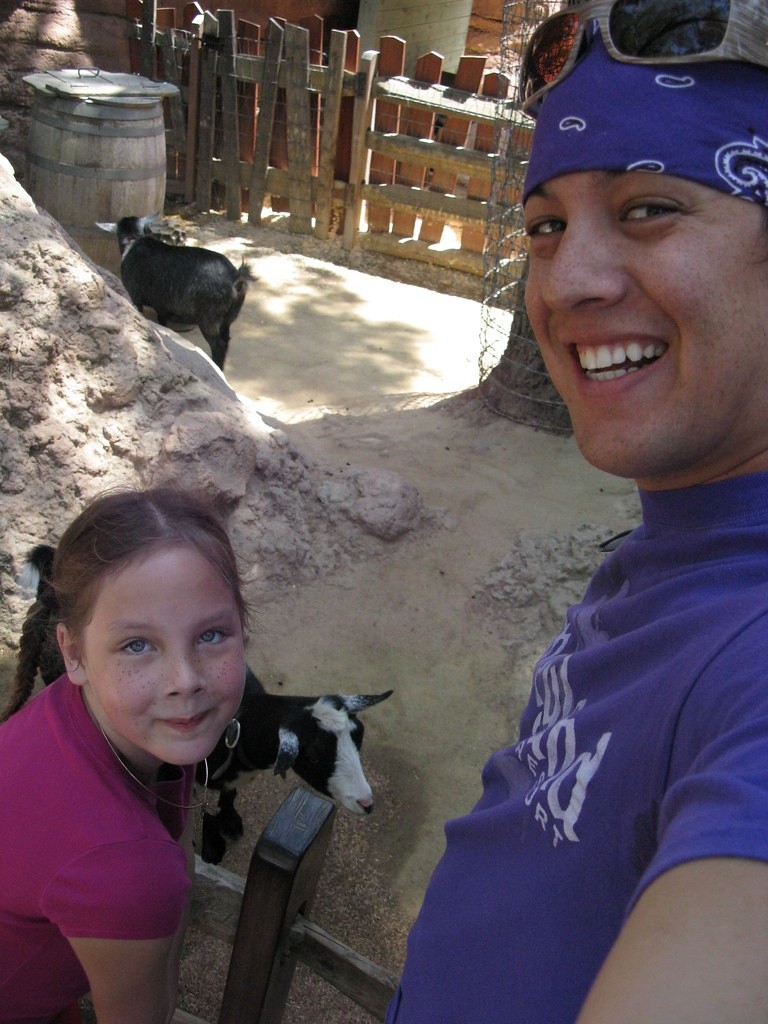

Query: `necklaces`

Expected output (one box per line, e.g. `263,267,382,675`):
98,724,210,849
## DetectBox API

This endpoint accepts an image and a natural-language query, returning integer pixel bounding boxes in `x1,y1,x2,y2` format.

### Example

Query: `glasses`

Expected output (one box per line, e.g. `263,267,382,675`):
523,0,768,122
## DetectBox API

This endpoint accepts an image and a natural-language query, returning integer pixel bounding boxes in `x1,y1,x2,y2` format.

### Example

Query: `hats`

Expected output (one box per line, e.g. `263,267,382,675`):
522,15,768,206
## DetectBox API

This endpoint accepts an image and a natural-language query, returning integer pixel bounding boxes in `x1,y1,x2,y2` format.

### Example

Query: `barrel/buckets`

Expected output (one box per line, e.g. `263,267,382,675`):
26,90,167,276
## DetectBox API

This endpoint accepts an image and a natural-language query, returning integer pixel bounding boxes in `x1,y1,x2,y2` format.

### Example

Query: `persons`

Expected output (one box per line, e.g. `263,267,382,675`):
391,1,768,1023
1,489,251,1023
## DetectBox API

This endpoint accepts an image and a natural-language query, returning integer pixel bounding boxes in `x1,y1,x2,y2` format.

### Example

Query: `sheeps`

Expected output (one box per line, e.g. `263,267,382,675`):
18,543,398,872
95,209,260,372
